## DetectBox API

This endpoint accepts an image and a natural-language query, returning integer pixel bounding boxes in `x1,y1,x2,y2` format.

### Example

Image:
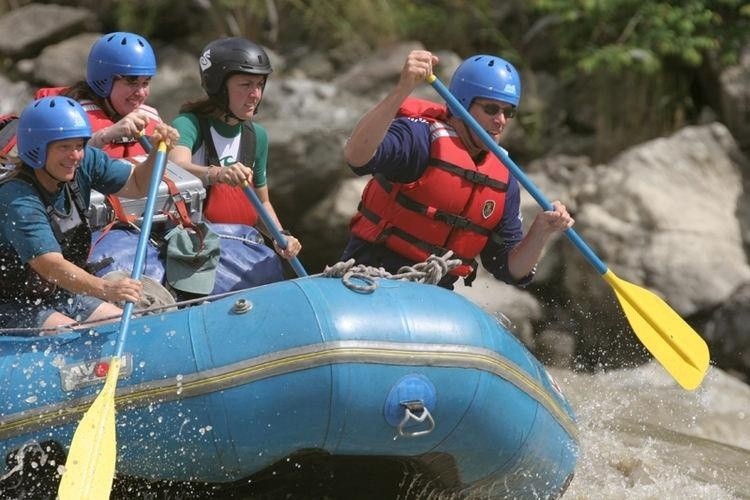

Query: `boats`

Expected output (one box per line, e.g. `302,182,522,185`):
0,267,583,500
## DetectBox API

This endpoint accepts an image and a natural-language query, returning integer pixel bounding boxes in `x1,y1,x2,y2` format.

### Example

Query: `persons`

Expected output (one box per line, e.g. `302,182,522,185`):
169,35,303,311
2,30,168,300
1,95,182,336
338,47,576,292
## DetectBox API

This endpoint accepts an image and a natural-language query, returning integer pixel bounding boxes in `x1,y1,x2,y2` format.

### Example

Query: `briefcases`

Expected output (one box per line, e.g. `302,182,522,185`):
84,153,209,232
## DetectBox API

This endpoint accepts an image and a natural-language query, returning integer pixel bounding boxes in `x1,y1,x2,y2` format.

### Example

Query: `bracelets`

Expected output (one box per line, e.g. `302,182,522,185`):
269,228,292,241
91,127,111,150
206,163,216,187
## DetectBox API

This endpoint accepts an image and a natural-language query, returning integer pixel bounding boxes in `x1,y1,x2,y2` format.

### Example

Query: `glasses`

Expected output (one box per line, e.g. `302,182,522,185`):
472,101,516,119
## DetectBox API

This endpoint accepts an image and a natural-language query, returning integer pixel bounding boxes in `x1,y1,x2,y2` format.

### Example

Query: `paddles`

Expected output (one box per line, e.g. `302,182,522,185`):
426,73,710,390
54,141,167,499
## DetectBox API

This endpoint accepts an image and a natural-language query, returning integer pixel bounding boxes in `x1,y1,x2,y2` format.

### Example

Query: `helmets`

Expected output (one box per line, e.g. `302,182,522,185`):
15,96,94,170
198,34,273,108
85,31,158,97
446,53,522,122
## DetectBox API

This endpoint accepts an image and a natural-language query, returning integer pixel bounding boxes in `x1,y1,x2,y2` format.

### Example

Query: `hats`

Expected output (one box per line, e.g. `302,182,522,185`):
164,218,222,296
